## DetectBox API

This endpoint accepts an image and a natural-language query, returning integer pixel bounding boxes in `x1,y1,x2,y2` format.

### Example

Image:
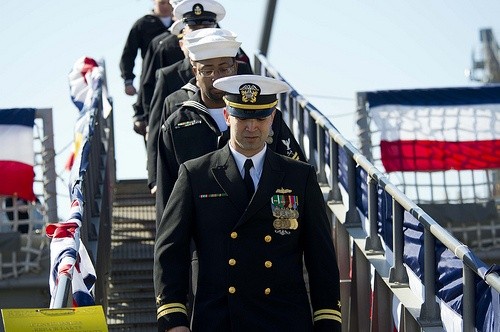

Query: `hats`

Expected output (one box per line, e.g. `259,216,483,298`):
212,74,290,118
184,27,241,61
173,0,226,28
170,20,185,38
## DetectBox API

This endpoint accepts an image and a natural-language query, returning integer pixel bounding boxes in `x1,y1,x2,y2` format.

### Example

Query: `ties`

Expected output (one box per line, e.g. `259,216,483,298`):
244,159,254,208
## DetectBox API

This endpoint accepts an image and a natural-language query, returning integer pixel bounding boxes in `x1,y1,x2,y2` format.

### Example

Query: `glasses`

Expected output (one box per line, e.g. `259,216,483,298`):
196,65,235,78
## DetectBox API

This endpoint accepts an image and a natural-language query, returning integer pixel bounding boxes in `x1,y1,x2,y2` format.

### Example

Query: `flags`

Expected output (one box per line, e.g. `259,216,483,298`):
365,83,500,207
0,107,37,203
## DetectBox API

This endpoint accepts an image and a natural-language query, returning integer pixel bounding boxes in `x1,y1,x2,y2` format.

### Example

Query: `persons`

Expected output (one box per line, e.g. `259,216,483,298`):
153,74,341,332
119,0,252,192
152,36,308,332
